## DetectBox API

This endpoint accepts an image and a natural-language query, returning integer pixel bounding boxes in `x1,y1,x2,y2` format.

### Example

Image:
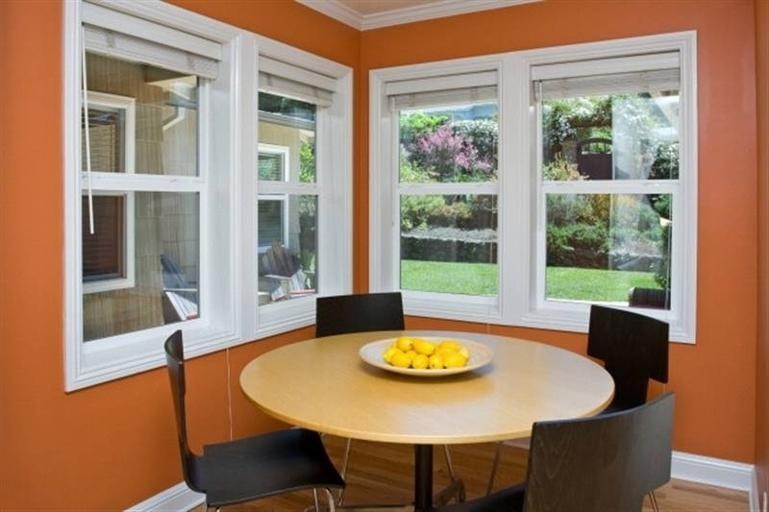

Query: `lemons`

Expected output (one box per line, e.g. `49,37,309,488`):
384,337,470,369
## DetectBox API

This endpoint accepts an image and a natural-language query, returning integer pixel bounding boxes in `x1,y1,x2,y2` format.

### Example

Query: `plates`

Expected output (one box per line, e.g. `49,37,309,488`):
358,338,494,377
